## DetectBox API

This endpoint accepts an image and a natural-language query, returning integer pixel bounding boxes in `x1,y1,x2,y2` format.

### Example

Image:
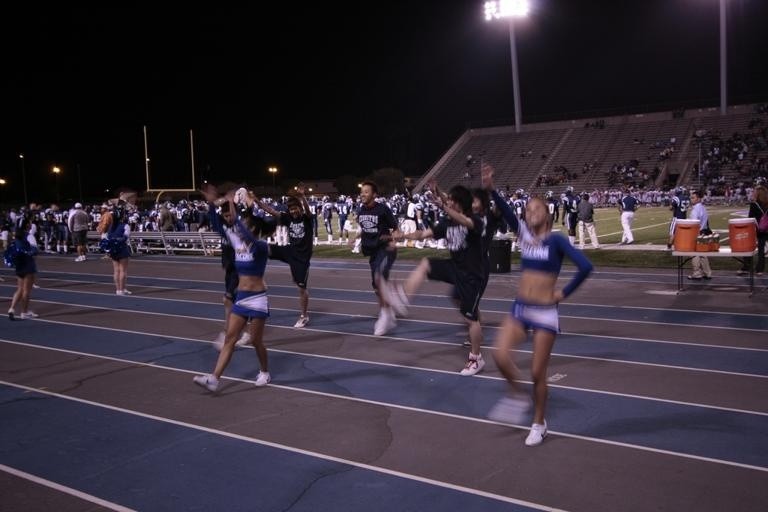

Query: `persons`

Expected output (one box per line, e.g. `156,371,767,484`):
0,106,768,447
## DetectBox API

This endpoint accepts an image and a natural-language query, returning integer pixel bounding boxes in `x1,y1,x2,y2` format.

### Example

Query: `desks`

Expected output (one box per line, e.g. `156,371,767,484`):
672,246,759,297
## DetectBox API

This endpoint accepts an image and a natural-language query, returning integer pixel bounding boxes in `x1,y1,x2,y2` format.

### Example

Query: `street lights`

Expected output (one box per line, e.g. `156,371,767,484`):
19,153,28,204
482,0,539,135
51,164,61,203
267,167,280,193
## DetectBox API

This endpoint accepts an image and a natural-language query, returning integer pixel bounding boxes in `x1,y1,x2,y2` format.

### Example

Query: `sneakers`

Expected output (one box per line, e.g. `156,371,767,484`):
525,420,548,447
75,255,85,261
116,288,131,295
8,309,15,320
461,352,485,376
255,370,271,387
374,309,396,335
382,281,409,315
294,315,309,329
194,375,219,392
21,311,38,319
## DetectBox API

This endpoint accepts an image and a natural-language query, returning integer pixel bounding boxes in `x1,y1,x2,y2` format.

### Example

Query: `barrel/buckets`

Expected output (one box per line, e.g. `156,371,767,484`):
727,217,758,252
674,219,701,253
